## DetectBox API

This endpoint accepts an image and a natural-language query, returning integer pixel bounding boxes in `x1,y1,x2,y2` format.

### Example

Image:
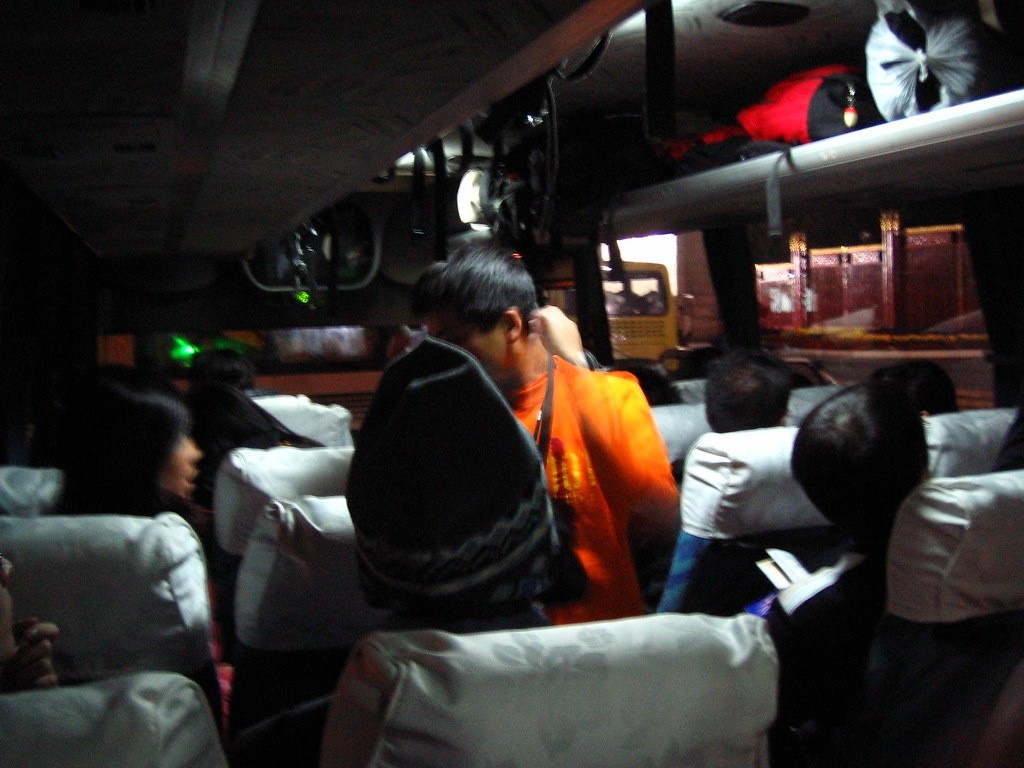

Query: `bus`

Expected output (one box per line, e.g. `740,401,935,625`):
544,260,677,364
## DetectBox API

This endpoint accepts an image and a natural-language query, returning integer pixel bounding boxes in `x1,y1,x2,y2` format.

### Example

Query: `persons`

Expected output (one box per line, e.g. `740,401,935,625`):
616,346,732,405
643,351,793,603
226,338,561,768
48,378,235,730
0,555,60,697
128,348,316,511
386,247,682,624
866,357,960,417
765,383,929,768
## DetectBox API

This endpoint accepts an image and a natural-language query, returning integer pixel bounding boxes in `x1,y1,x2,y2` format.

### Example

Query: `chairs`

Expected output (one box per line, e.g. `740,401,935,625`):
0,376,1024,768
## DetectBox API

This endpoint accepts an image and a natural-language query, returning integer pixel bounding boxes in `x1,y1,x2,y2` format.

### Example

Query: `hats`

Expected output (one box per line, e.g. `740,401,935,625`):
346,332,561,622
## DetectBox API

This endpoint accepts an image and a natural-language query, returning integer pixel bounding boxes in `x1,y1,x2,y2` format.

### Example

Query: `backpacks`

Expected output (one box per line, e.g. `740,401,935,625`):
648,64,882,236
